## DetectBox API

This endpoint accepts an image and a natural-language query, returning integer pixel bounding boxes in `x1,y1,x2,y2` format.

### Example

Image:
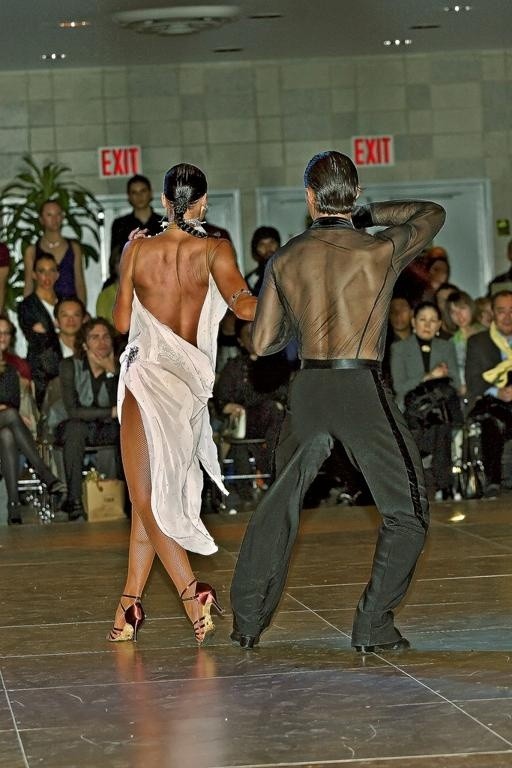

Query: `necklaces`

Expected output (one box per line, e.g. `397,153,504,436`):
45,235,60,248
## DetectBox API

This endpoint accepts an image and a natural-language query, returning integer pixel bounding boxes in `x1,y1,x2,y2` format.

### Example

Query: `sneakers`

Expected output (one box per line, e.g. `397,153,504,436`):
485,486,499,501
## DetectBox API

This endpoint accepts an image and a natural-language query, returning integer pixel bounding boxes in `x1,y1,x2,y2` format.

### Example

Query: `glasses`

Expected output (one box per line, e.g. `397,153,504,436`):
0,328,11,336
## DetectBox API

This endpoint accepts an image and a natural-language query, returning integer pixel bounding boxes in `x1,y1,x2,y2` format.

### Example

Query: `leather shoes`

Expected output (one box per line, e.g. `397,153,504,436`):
230,633,259,650
69,498,87,522
8,502,23,525
36,474,67,495
357,638,411,655
441,486,455,501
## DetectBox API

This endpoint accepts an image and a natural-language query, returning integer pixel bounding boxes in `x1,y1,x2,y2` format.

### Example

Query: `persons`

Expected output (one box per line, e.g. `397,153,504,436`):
45,317,132,525
0,242,11,314
18,253,91,359
111,174,169,247
214,318,289,489
464,292,512,499
97,246,123,328
382,296,414,384
485,239,512,295
229,151,445,655
106,164,259,647
391,301,461,501
446,291,487,396
0,317,67,526
220,226,281,335
32,298,84,413
410,247,450,301
474,298,494,329
434,283,460,313
23,201,87,308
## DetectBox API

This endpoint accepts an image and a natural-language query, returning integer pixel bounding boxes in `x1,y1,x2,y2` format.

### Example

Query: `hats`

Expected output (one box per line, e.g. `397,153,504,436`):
252,226,281,251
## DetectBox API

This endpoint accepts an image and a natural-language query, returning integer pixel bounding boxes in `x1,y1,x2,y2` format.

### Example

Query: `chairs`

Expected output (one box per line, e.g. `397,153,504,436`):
42,377,121,524
14,378,42,524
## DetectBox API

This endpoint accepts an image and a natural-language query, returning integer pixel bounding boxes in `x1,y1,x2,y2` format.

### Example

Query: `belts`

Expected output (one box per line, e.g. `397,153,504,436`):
299,359,381,368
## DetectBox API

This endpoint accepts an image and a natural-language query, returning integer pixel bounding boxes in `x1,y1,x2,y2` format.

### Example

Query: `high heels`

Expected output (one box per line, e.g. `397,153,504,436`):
107,595,144,642
180,578,225,649
